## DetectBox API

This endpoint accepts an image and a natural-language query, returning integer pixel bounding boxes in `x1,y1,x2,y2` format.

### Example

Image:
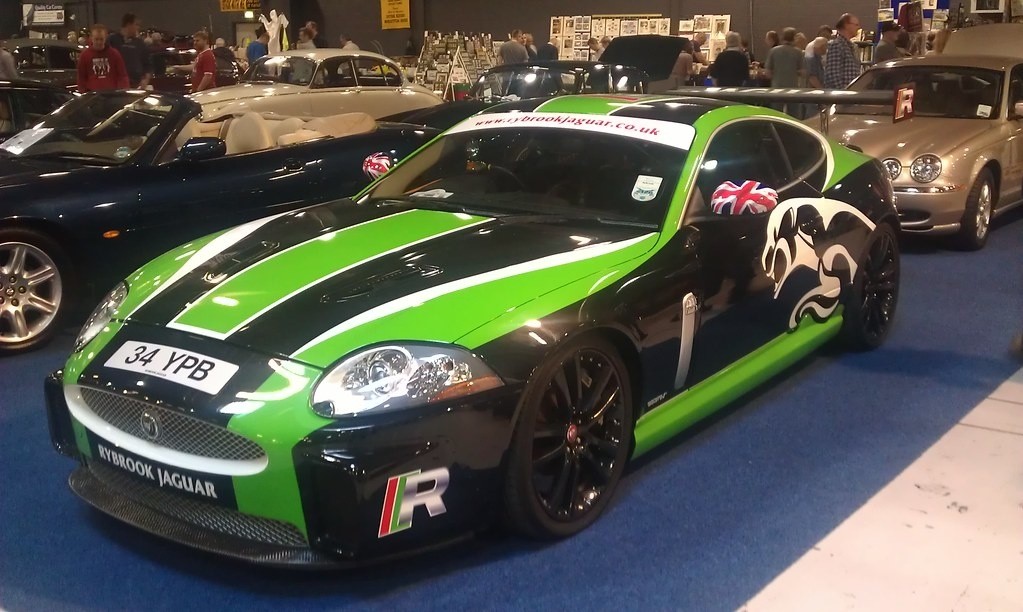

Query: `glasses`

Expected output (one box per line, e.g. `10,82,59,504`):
846,22,859,27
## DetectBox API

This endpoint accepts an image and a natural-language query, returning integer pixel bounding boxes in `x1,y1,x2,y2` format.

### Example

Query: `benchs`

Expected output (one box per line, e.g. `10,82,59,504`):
271,117,337,145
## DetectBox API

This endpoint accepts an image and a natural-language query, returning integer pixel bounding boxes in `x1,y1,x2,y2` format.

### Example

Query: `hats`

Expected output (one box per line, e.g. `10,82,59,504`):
882,21,900,33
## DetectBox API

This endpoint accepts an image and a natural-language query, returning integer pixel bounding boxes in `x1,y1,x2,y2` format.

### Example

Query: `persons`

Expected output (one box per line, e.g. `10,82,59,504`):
824,12,861,88
211,38,235,62
690,32,707,63
672,41,698,86
190,31,217,93
751,25,833,88
405,36,420,57
587,36,611,61
872,20,911,63
46,12,176,93
497,29,558,97
292,22,329,84
337,31,359,77
925,29,953,56
711,31,756,87
247,34,270,80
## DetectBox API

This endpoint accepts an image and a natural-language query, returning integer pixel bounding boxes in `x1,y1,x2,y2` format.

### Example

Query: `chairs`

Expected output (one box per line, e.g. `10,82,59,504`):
175,118,200,152
323,74,345,88
226,111,274,155
936,80,965,113
909,78,941,113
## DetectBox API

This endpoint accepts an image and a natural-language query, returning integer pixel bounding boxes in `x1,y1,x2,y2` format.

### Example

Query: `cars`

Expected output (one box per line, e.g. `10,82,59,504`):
0,88,449,355
373,35,693,151
186,50,445,127
0,38,87,114
151,47,244,93
798,22,1023,250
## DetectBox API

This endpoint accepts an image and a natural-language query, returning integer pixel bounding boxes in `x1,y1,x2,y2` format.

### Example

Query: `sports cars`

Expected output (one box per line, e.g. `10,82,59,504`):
47,91,903,591
